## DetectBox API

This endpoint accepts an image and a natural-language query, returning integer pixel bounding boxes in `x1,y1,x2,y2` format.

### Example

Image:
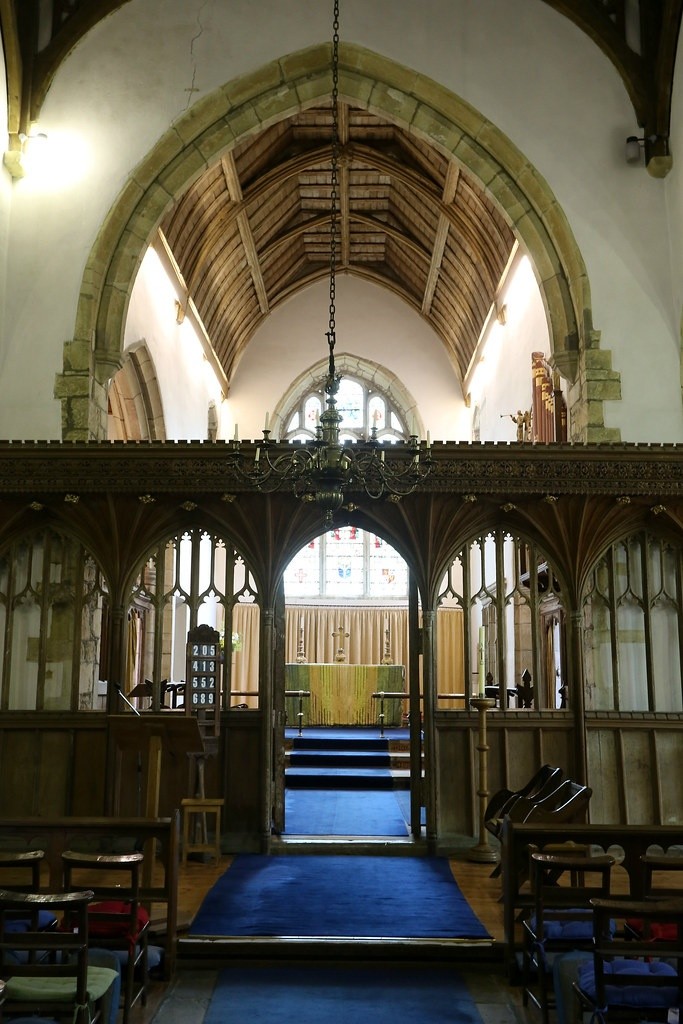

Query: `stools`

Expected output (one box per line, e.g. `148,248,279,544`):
181,799,224,867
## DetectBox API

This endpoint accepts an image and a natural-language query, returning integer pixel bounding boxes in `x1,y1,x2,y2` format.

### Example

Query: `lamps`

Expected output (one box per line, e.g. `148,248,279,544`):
229,2,439,528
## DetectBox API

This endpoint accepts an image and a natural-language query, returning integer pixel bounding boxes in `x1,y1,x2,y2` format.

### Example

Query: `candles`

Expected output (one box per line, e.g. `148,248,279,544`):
478,625,485,695
300,616,305,629
385,618,390,631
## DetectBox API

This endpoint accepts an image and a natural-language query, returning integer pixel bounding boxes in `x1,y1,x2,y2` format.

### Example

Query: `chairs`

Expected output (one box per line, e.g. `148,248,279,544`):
528,854,682,1024
0,852,152,1023
